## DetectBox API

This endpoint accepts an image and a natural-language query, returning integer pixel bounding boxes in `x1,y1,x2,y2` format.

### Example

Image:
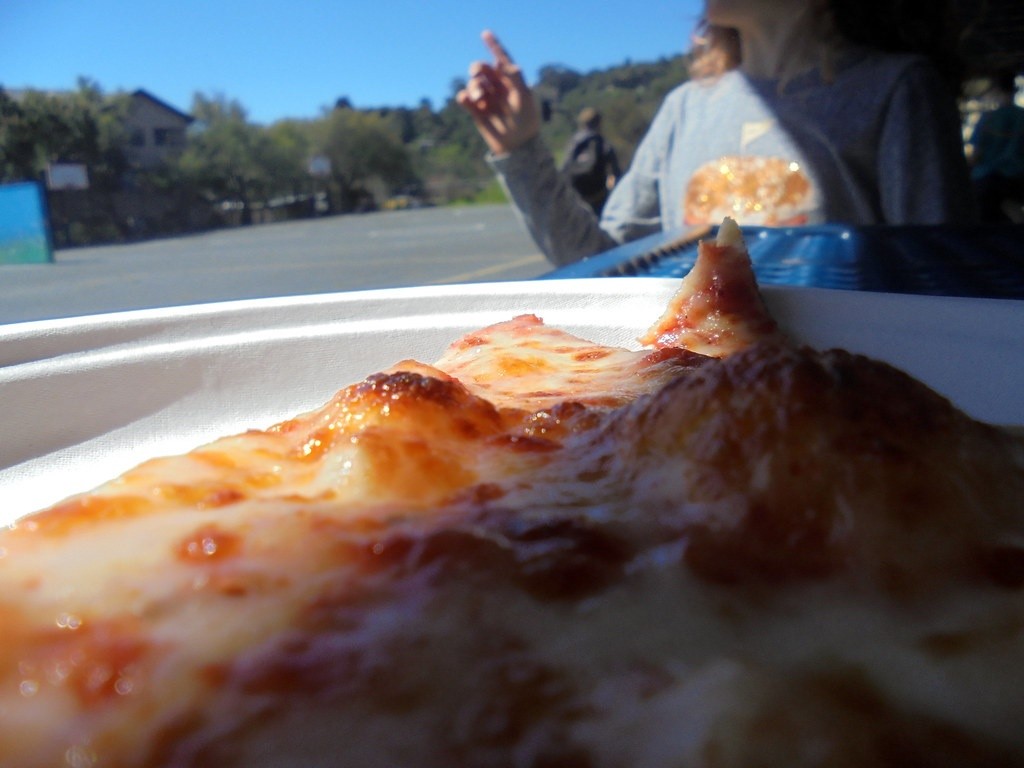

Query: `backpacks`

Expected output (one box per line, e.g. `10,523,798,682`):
563,134,606,195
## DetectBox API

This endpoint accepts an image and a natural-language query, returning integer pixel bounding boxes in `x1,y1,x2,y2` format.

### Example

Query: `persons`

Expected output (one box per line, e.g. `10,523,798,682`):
457,0,969,268
559,105,621,222
962,73,1024,224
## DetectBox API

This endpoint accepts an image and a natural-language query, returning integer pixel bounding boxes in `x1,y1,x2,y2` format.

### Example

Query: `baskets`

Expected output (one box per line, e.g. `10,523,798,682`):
532,224,855,291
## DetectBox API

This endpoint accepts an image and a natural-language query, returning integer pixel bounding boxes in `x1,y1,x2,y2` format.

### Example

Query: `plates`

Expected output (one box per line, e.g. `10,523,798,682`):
0,276,1024,768
